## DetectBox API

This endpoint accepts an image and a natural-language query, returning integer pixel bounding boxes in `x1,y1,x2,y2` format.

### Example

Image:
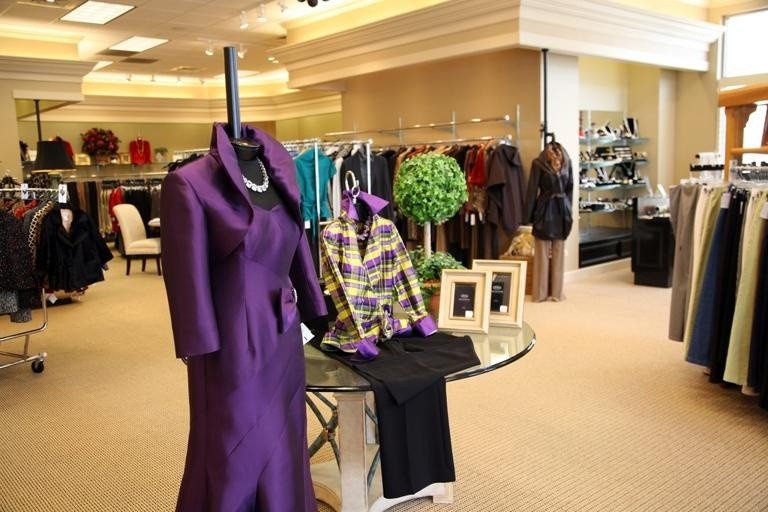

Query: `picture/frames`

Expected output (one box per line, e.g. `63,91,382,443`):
438,269,490,335
472,260,527,328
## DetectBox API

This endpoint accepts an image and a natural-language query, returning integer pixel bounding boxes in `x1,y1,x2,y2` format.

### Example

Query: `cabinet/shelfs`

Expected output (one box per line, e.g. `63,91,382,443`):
579,110,647,269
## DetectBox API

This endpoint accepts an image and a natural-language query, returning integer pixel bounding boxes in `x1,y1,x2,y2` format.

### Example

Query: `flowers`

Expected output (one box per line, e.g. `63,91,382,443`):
80,128,119,154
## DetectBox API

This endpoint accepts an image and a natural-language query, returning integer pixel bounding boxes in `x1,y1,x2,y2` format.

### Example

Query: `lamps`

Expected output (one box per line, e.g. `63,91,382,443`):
32,142,77,189
239,11,249,29
277,3,290,15
205,42,215,55
254,4,267,23
236,42,248,58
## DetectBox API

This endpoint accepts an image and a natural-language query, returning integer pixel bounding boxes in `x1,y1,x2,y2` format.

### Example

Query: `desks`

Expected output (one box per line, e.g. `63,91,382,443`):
306,317,536,512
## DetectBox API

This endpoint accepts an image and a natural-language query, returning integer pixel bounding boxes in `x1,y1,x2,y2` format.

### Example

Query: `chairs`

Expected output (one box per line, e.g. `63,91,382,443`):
114,204,162,276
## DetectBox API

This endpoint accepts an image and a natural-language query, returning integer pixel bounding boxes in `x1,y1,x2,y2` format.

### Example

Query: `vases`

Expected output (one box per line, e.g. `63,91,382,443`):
90,151,111,165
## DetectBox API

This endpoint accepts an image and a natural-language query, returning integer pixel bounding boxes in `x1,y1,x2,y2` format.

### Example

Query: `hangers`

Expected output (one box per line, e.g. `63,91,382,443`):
320,139,373,196
1,189,61,373
282,138,324,278
